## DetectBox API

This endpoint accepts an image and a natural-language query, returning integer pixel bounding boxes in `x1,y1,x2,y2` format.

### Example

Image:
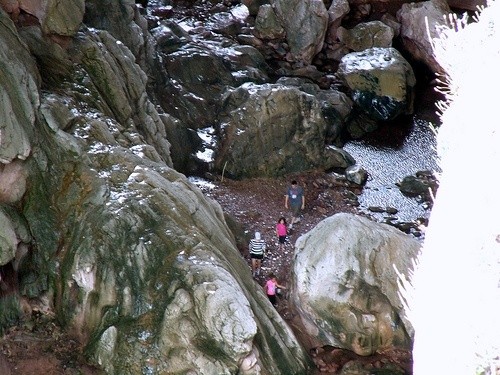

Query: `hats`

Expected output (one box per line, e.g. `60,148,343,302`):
255,232,261,240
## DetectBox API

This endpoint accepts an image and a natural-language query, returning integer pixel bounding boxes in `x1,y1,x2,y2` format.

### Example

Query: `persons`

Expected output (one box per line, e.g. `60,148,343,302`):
263,276,286,307
285,180,305,228
248,232,266,277
276,217,289,251
